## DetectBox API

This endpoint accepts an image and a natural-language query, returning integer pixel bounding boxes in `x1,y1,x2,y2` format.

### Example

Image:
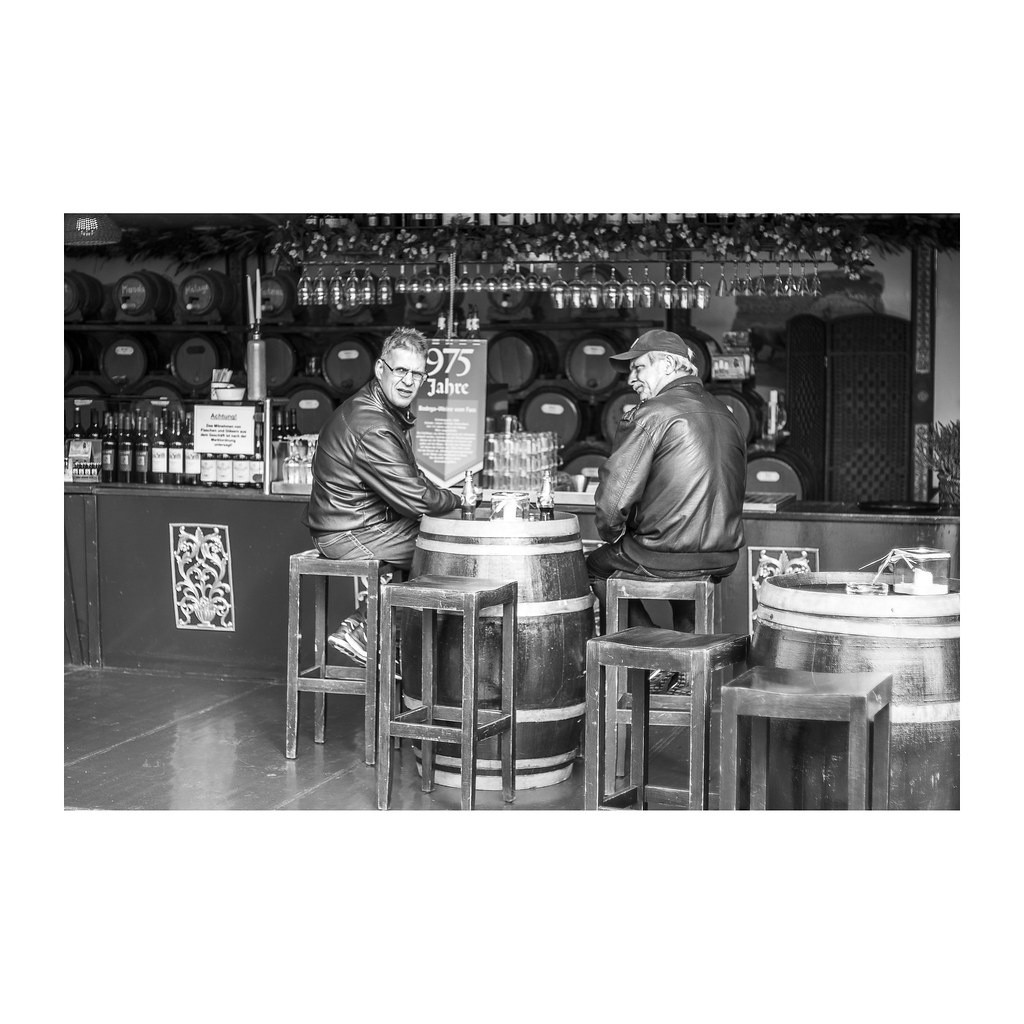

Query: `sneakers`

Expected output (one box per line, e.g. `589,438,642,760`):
345,620,403,680
327,618,366,667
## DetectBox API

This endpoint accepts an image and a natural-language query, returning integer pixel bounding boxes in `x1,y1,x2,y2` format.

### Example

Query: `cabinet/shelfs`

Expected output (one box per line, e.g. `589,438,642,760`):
64,319,659,462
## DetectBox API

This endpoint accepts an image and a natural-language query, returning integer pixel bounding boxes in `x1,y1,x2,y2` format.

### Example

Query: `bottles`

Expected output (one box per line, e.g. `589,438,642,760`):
538,476,556,520
64,402,302,492
459,471,479,520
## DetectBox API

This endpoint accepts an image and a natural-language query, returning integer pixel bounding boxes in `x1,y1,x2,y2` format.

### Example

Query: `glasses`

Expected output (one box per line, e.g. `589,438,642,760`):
380,358,427,381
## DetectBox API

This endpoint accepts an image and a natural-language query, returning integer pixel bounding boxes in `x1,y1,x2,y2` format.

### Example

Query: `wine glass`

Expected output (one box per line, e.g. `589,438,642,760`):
281,432,320,485
297,260,823,311
482,431,558,493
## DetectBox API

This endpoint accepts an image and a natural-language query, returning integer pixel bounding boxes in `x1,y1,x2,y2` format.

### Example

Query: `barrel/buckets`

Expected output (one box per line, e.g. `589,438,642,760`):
483,265,816,499
65,265,457,434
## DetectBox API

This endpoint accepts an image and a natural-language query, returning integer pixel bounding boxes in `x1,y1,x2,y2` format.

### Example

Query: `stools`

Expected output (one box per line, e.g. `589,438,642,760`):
604,570,722,795
719,665,893,810
378,574,519,811
583,626,750,811
285,548,404,764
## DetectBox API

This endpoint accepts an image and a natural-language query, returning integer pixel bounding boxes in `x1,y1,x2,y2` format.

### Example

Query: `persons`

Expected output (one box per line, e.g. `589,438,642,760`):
302,328,461,679
586,330,748,694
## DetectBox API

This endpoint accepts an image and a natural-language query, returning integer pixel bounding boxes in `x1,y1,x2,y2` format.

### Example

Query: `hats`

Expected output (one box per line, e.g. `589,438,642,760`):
609,330,690,373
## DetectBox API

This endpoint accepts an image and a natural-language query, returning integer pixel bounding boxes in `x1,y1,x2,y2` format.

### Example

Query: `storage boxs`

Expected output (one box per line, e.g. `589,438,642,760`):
893,545,951,595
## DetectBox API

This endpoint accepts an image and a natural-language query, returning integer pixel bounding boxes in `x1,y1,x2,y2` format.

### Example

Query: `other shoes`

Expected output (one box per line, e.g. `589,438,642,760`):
650,670,675,694
667,671,691,696
